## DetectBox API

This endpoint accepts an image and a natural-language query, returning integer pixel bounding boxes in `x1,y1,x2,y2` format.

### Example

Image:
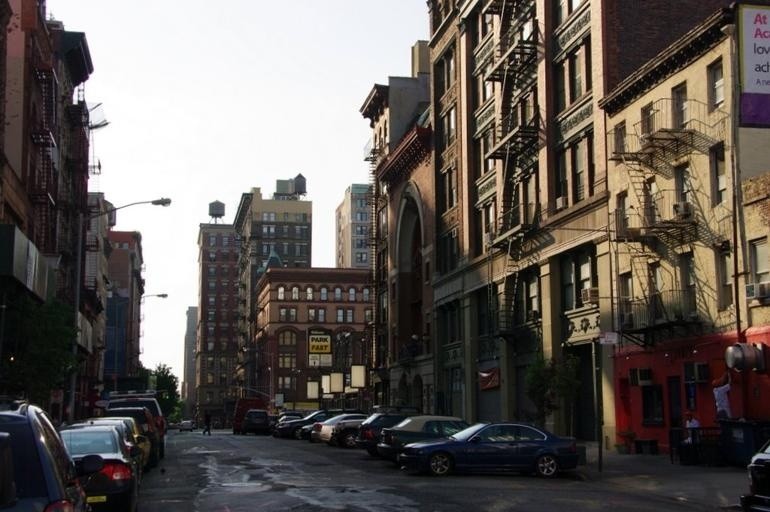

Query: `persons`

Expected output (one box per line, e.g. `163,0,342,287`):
201,409,211,435
711,370,733,445
682,409,700,446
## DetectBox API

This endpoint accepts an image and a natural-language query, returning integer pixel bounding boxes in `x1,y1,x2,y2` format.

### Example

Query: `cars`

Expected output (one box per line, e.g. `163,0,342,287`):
740,435,770,512
0,385,194,511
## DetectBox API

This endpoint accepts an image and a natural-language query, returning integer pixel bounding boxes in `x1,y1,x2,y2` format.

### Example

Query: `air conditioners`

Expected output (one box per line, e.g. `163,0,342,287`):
745,282,769,300
673,201,691,217
451,228,460,238
555,196,568,210
580,287,600,304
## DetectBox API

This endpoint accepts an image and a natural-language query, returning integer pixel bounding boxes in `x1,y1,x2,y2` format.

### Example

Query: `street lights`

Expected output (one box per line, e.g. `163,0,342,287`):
114,291,169,392
68,199,170,424
